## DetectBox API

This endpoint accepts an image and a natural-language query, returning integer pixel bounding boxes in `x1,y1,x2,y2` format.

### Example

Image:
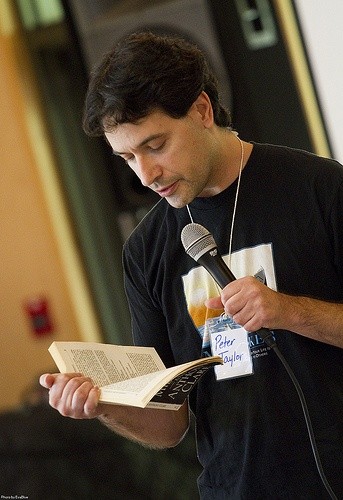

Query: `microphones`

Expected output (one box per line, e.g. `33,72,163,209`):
181,224,277,348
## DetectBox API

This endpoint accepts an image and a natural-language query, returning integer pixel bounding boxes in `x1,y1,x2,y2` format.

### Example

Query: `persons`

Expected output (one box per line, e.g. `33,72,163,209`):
38,31,342,500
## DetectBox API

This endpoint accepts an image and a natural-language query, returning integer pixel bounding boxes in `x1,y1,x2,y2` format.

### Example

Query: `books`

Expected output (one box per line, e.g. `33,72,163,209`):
46,340,224,412
